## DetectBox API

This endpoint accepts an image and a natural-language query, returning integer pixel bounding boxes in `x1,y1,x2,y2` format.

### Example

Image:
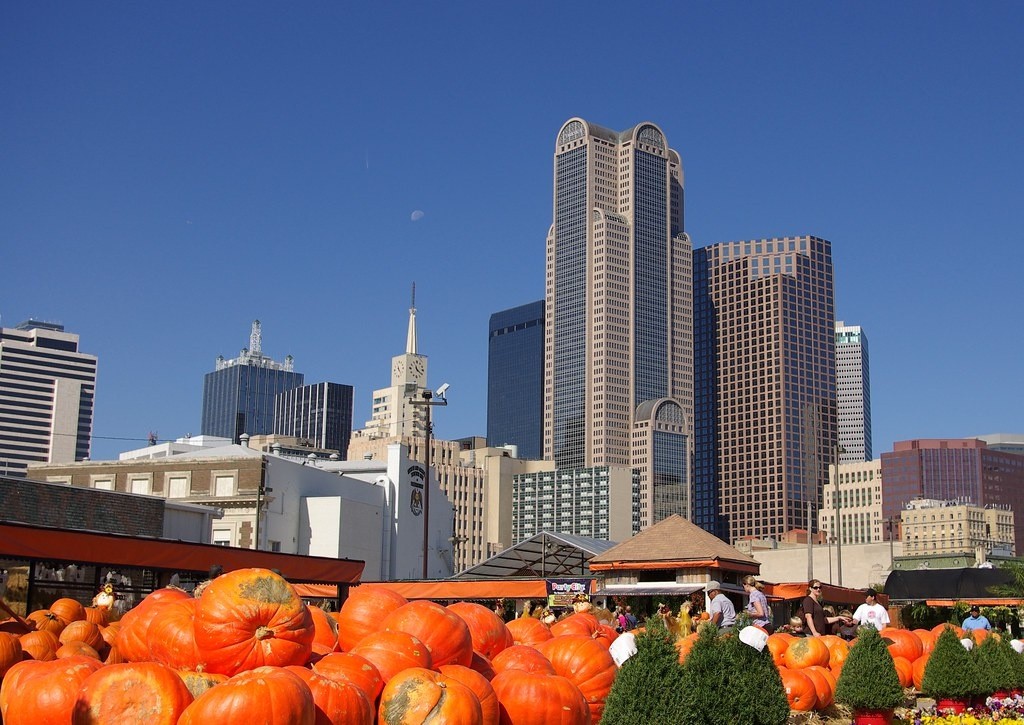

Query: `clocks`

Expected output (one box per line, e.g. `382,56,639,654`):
409,360,424,379
393,361,404,378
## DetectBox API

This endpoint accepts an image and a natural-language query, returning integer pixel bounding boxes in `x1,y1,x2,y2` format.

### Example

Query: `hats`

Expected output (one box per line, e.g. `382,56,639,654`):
971,605,979,611
864,589,877,596
702,581,720,592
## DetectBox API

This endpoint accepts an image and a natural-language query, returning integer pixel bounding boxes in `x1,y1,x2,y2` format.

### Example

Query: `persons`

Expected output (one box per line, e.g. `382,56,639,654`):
962,607,992,632
92,565,222,612
495,576,891,643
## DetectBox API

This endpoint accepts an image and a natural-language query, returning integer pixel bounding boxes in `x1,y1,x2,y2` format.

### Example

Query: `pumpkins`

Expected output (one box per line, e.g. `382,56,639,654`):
0,567,1024,725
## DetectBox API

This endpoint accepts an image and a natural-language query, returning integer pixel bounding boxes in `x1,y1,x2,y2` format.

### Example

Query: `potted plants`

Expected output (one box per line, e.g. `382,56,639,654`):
966,636,996,712
920,621,983,715
834,620,907,725
999,637,1024,699
979,633,1017,699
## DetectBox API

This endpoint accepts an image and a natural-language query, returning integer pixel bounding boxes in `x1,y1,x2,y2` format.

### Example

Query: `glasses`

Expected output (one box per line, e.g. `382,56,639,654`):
708,590,714,595
811,586,822,589
